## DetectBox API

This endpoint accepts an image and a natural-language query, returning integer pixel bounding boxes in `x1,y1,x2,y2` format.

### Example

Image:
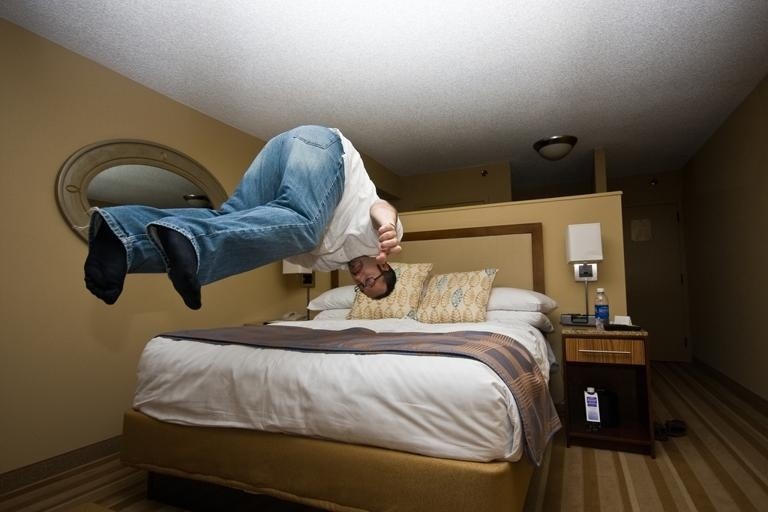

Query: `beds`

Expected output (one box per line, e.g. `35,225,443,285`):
114,221,561,512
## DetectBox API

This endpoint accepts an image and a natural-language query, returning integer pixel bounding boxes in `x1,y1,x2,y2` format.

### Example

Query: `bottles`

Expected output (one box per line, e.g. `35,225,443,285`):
593,287,609,330
583,386,603,433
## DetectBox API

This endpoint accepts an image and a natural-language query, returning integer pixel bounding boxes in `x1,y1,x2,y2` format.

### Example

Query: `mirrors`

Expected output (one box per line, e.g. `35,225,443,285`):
51,136,229,250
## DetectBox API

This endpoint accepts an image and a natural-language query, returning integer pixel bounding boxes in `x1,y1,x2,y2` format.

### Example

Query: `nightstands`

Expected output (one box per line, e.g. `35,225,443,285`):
558,325,654,462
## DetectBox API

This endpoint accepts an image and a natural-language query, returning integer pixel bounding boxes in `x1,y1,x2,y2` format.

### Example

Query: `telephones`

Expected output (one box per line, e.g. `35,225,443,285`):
282,312,307,321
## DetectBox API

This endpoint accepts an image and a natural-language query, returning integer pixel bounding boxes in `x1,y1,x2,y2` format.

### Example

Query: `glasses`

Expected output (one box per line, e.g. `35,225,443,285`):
353,272,383,295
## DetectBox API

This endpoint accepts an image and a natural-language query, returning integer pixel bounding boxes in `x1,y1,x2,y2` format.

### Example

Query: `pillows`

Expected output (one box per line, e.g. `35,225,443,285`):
309,307,353,321
413,267,500,323
484,286,559,315
305,284,358,311
344,260,435,320
482,309,555,335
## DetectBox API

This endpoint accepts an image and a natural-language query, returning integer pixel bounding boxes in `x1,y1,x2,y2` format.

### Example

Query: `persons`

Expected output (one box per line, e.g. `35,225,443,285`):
84,124,404,309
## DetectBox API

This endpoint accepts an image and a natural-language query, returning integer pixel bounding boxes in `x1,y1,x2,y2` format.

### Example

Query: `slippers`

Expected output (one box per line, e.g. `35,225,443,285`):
653,419,689,441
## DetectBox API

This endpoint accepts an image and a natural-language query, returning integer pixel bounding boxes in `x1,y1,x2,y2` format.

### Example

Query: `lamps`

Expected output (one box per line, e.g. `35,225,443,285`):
280,257,313,319
561,221,605,327
531,133,577,162
